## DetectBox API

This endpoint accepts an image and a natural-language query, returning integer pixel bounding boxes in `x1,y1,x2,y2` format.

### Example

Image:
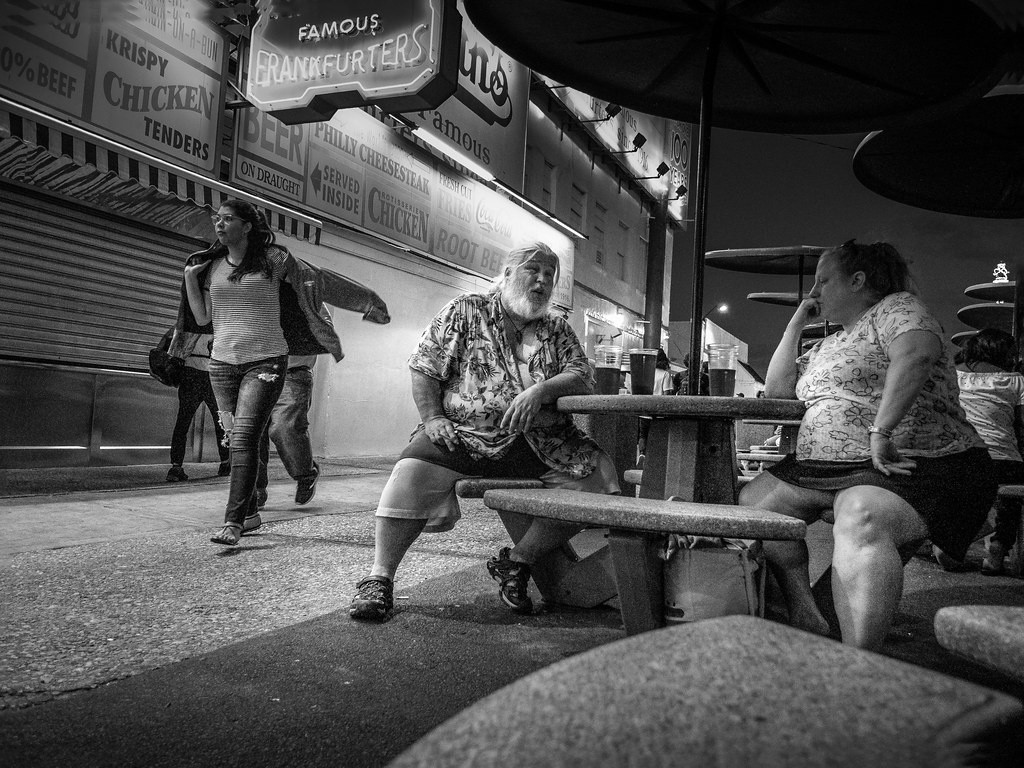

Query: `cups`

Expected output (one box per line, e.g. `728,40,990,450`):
627,348,659,395
707,344,739,397
593,345,623,395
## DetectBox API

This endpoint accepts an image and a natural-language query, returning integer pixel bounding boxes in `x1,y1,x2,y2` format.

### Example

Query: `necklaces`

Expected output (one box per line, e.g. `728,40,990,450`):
500,299,531,345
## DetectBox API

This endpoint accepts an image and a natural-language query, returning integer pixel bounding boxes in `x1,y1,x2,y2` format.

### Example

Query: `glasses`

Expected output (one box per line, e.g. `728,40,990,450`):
840,238,873,266
211,214,246,226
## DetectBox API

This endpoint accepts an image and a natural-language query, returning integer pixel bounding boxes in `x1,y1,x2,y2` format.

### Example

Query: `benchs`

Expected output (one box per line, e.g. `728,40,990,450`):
388,446,1024,768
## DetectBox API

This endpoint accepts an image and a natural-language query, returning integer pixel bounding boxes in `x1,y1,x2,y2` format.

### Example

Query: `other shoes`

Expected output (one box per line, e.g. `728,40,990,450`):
217,462,231,476
257,490,268,509
165,466,189,481
294,460,321,505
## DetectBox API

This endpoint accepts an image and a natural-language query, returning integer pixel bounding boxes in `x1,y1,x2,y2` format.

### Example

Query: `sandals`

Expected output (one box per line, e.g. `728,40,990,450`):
349,576,393,619
486,547,534,613
210,524,244,545
243,515,262,535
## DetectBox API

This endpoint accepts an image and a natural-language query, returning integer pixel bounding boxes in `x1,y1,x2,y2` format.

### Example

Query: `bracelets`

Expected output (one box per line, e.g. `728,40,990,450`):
867,422,893,438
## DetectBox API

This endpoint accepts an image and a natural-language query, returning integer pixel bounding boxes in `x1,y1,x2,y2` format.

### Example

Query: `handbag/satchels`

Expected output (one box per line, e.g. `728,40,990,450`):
149,348,184,389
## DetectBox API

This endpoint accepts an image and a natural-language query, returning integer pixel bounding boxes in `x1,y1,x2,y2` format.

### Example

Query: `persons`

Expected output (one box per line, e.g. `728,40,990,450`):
255,302,334,512
348,240,622,619
636,348,783,470
737,239,998,653
174,200,391,546
165,328,231,481
953,328,1024,572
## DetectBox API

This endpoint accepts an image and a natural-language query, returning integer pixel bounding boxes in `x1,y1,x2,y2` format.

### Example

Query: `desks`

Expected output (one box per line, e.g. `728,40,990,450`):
558,396,807,505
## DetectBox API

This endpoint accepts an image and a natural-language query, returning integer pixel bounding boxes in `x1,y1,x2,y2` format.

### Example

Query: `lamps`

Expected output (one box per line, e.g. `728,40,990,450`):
618,161,670,194
561,102,622,142
641,185,687,213
592,133,647,169
